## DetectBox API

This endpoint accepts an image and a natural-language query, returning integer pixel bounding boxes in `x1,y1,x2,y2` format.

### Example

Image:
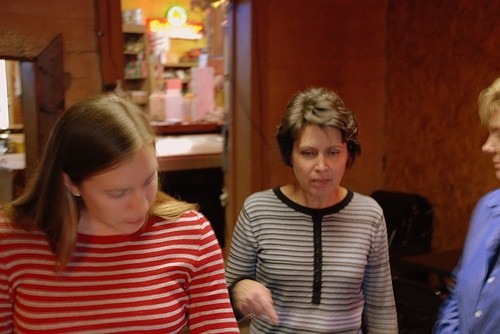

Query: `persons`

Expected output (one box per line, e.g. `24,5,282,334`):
0,93,239,333
225,89,400,333
433,78,500,334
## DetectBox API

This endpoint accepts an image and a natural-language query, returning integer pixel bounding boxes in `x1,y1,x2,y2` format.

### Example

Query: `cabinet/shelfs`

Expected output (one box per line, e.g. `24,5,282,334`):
122,23,221,133
